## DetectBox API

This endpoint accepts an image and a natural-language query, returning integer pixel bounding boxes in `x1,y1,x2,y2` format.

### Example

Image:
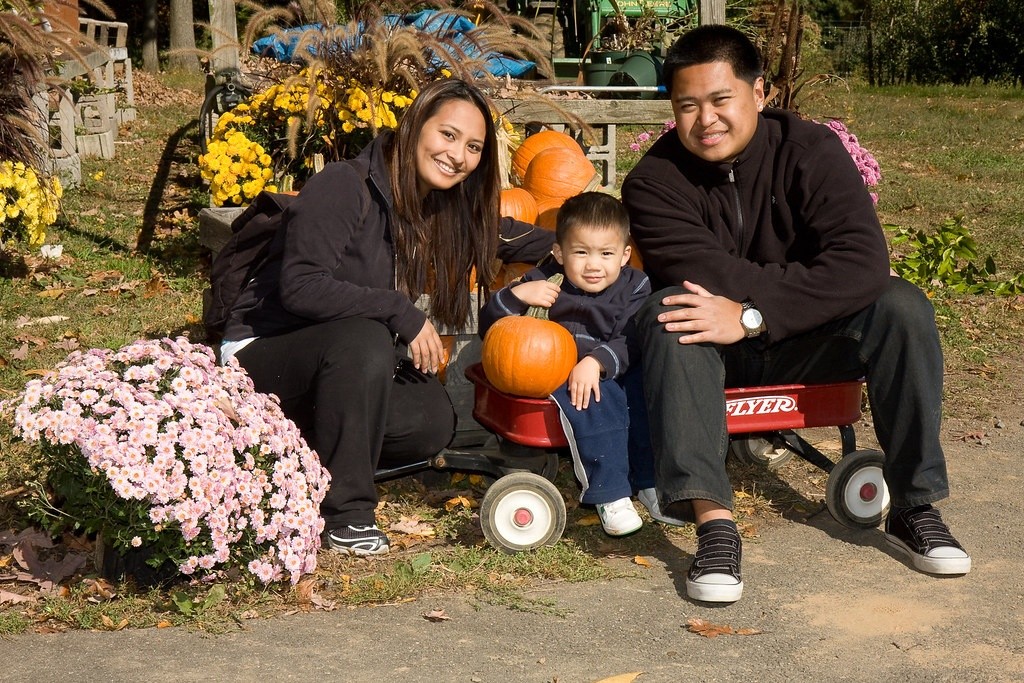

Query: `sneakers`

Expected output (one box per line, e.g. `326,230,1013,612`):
328,525,391,556
686,519,742,602
885,503,971,575
595,497,642,534
637,486,684,525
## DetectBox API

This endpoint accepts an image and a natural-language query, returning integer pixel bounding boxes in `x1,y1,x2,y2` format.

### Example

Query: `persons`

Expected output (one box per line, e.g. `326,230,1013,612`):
623,25,973,603
218,77,559,560
478,191,693,537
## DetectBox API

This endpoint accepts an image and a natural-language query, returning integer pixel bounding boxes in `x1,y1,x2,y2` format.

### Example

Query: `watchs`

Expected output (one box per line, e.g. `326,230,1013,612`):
740,301,762,338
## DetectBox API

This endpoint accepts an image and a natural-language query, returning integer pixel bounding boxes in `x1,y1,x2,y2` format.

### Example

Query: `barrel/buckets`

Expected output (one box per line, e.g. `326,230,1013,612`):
579,48,664,99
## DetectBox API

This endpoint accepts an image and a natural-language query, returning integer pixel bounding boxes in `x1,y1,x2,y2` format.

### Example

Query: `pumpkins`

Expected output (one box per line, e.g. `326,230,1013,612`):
481,274,579,398
434,132,644,384
